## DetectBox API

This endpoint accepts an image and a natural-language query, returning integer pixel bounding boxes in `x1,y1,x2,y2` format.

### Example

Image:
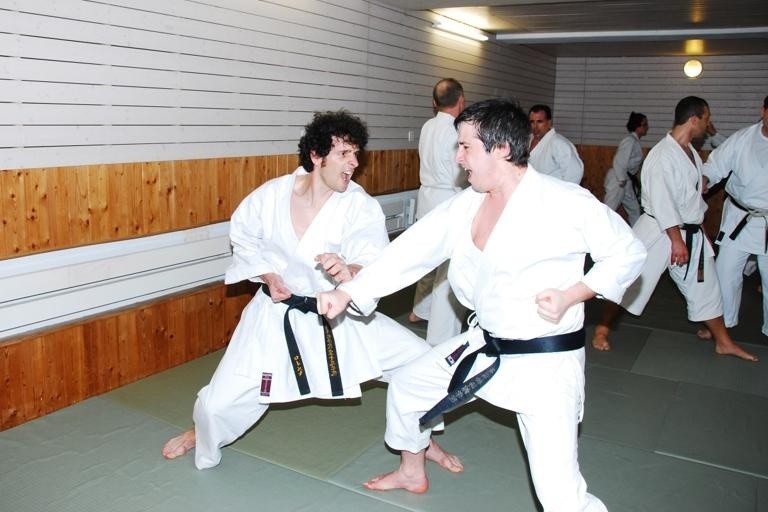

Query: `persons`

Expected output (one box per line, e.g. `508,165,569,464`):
697,95,768,340
161,109,464,474
316,97,650,511
407,75,472,349
600,110,648,228
523,102,585,188
590,96,759,362
706,121,727,148
